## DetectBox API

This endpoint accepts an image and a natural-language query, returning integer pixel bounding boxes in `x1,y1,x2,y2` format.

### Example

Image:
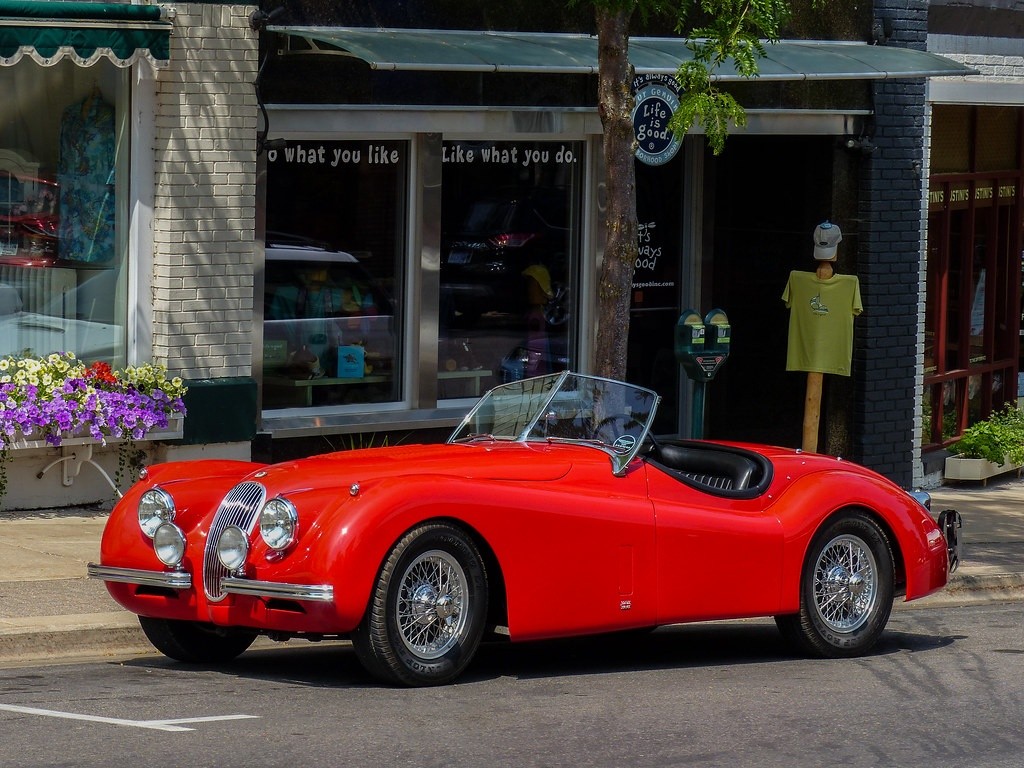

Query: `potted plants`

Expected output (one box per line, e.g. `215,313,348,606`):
945,399,1024,486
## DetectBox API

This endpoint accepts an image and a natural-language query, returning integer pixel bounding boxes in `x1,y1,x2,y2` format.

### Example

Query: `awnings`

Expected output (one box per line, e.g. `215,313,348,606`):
265,26,980,85
0,0,174,72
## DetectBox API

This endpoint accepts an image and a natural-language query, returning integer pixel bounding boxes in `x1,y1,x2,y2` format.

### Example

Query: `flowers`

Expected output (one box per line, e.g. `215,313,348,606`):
0,351,187,503
8,188,58,216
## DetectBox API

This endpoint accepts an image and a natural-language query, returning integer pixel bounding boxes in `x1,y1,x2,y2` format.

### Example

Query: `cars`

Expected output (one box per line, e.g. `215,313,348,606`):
440,198,575,329
0,171,59,265
497,335,576,392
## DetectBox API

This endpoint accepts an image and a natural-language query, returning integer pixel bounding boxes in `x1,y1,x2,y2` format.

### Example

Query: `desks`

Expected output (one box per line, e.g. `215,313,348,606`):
263,369,492,407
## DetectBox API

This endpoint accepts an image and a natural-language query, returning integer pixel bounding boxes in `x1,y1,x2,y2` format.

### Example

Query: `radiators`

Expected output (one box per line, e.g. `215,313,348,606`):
0,265,76,319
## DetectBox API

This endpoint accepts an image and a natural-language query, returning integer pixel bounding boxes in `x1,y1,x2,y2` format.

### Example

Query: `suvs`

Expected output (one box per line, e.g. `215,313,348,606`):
257,229,449,379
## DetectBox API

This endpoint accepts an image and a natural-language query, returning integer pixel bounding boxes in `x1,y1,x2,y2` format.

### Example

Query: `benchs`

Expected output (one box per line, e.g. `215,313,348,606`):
648,443,756,491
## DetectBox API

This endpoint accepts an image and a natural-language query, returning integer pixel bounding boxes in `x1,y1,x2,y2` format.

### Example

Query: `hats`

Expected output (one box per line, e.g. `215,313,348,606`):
522,265,553,296
814,223,842,259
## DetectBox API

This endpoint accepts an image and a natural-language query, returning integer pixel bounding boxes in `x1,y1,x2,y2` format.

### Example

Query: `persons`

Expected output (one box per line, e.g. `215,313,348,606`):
265,256,397,406
512,222,674,356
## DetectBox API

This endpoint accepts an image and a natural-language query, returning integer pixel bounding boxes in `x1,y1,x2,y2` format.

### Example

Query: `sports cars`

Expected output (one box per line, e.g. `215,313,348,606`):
88,371,963,689
